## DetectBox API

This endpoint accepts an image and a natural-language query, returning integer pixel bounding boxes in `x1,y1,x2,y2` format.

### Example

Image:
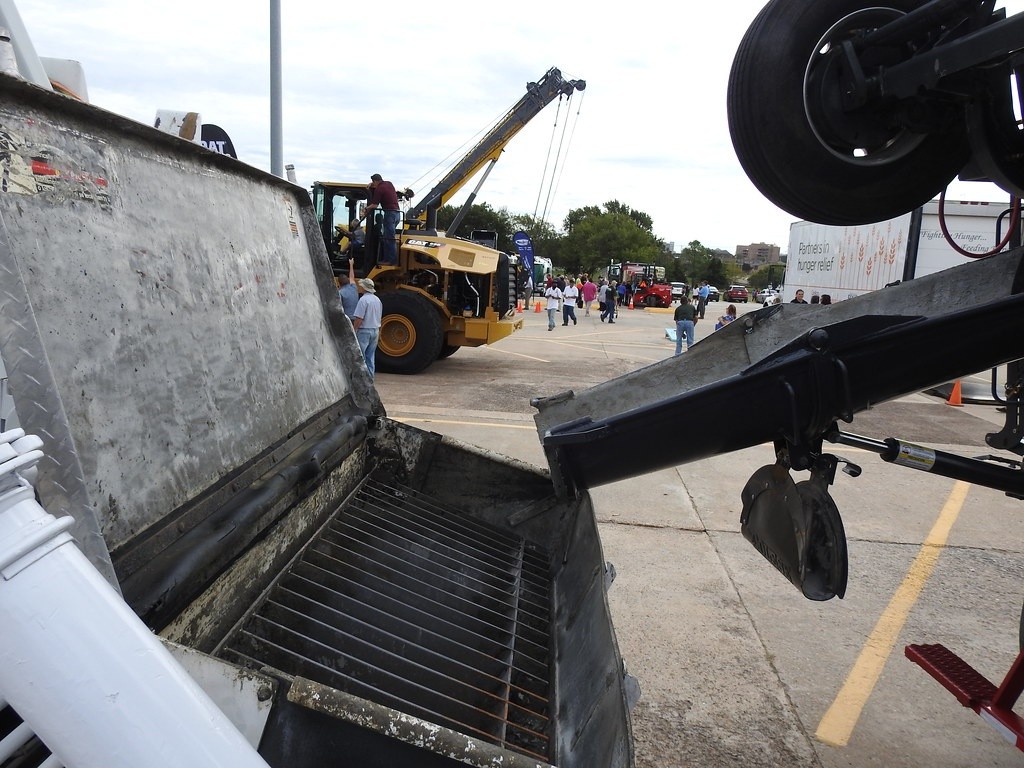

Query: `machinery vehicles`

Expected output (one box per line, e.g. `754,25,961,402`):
311,65,586,375
604,258,672,308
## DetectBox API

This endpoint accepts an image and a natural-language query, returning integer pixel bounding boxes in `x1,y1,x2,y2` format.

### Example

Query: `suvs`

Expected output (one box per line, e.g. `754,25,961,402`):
723,285,748,303
671,282,684,300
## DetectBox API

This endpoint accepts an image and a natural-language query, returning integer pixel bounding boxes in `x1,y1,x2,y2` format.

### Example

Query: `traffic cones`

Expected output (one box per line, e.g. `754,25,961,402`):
628,297,634,309
945,378,963,406
534,301,541,313
516,303,523,314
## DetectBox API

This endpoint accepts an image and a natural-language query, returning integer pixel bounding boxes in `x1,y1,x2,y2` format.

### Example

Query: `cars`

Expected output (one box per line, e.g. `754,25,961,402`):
708,286,719,302
756,289,777,303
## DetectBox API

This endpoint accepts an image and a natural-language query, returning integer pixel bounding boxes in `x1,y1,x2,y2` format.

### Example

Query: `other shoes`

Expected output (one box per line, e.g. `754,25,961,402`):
609,320,615,323
601,314,604,322
548,325,555,331
574,320,576,325
562,321,568,326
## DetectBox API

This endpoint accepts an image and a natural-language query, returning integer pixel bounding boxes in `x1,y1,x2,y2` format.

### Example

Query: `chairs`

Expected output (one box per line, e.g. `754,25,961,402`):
348,213,383,262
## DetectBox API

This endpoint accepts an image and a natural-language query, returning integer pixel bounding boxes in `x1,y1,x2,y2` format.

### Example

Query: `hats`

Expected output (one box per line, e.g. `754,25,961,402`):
559,275,564,278
552,280,557,283
352,219,360,226
701,280,708,284
578,274,582,278
612,281,617,286
358,277,376,293
584,273,588,276
338,274,348,284
570,278,575,282
681,296,688,303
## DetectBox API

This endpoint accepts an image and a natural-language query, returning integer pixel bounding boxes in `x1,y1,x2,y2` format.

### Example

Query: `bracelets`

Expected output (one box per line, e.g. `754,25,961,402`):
365,207,367,210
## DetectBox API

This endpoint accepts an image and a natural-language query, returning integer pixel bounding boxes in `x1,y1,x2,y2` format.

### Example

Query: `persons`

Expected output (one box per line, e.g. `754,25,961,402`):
820,294,832,305
523,276,533,310
361,173,400,266
790,289,808,304
338,258,359,324
810,295,820,304
334,219,366,259
353,277,383,377
682,280,759,326
674,296,698,355
544,273,632,331
